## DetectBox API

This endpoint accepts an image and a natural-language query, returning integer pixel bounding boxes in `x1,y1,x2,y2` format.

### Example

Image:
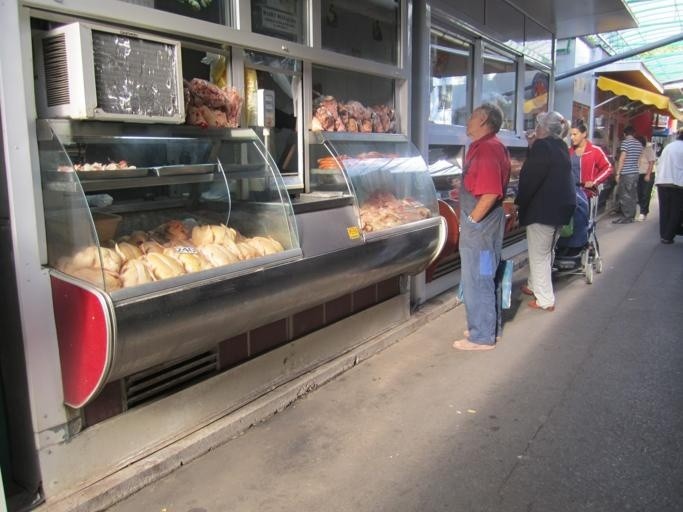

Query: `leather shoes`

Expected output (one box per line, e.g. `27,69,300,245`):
661,237,675,243
529,300,556,311
521,286,534,295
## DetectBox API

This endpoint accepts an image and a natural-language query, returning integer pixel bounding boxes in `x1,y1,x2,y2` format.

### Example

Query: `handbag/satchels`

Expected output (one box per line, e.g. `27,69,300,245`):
456,259,513,309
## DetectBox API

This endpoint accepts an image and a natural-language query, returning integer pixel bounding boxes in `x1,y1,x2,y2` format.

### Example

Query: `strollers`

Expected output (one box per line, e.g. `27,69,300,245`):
552,184,603,285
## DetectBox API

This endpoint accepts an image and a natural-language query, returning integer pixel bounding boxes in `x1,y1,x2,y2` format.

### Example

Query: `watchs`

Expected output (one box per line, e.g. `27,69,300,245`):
615,174,620,176
467,216,475,224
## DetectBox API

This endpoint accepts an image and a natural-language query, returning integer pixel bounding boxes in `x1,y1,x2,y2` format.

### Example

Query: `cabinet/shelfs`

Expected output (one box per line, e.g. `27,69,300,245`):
39,119,448,409
424,132,532,289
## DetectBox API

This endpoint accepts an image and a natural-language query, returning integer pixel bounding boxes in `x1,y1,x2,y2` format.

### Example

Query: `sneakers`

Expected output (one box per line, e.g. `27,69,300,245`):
638,214,649,222
463,329,501,342
618,218,633,224
453,340,495,351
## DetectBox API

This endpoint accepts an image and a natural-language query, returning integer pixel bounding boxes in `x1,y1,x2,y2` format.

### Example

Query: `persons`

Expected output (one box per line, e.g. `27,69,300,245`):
453,103,510,350
635,137,657,221
567,119,612,256
510,111,577,311
612,126,642,224
654,126,682,244
